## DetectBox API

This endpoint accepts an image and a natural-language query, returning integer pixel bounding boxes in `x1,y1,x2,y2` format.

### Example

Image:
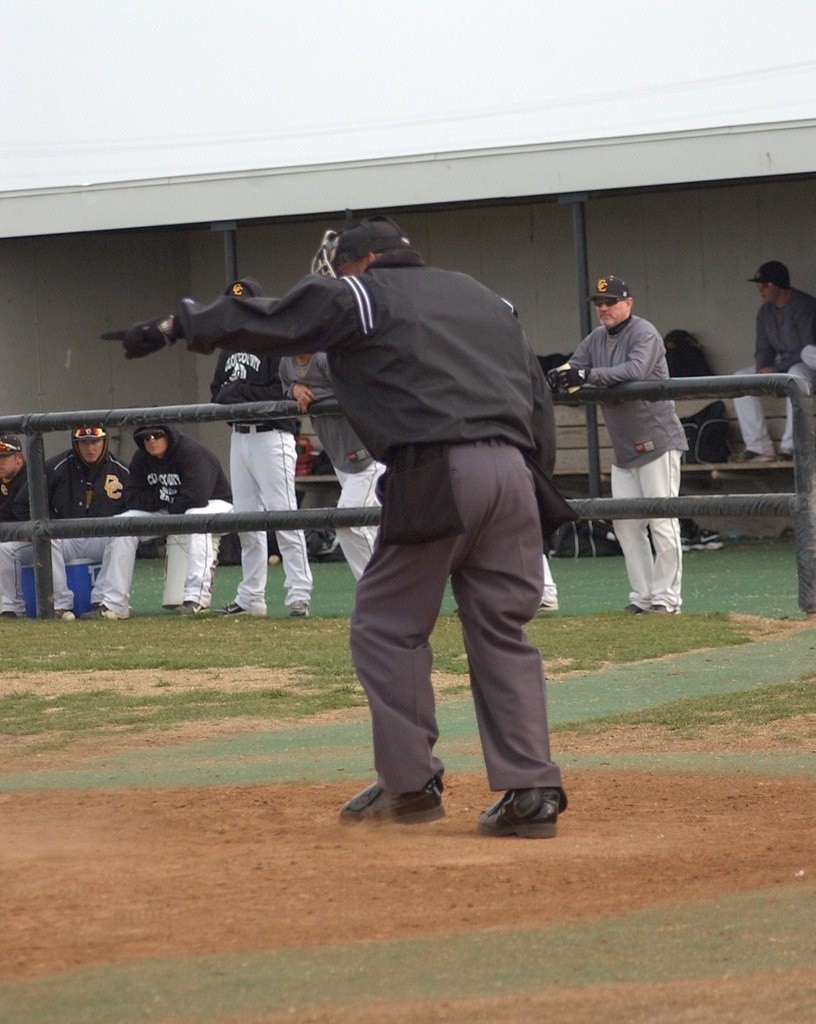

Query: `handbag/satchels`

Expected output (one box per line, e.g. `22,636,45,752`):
379,442,466,543
302,523,346,564
548,517,625,557
676,400,731,466
521,452,580,535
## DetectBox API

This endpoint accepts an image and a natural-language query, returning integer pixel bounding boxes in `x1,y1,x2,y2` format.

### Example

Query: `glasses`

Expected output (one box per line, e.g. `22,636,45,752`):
597,297,624,308
0,427,165,452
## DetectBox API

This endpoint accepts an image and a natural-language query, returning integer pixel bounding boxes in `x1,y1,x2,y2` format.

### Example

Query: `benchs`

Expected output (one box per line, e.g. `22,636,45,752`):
294,397,795,507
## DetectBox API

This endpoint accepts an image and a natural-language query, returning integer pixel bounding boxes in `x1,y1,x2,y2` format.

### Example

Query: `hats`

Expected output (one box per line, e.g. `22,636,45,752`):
325,220,409,273
228,284,251,299
746,260,789,288
0,428,167,456
590,276,631,300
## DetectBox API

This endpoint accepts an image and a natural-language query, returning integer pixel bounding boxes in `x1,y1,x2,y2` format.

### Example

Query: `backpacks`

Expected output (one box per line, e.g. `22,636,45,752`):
663,329,715,378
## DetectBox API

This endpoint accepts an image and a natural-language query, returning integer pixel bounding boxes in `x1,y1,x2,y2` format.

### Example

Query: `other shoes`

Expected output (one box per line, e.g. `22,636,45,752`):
536,603,681,614
727,450,775,463
775,453,793,462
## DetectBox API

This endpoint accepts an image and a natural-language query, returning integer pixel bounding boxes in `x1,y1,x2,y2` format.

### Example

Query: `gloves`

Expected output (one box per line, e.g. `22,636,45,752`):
100,314,174,360
546,368,561,394
558,368,590,390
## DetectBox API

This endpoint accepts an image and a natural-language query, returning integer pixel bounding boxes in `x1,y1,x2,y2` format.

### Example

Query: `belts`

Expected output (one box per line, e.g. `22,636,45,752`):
234,424,279,433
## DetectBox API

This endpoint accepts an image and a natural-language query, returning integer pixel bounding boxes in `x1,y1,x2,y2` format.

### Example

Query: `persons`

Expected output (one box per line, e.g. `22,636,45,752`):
728,261,816,462
548,275,689,615
454,540,561,613
278,351,388,582
13,419,131,620
210,275,314,618
100,213,568,838
80,421,234,619
0,433,34,622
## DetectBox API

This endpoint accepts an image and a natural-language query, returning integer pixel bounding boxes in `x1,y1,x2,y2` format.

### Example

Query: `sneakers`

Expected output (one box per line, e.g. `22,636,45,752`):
680,536,692,551
0,599,309,621
690,529,725,551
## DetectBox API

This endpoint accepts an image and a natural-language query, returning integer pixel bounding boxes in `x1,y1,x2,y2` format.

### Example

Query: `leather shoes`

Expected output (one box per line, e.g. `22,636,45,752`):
479,786,559,838
340,781,444,825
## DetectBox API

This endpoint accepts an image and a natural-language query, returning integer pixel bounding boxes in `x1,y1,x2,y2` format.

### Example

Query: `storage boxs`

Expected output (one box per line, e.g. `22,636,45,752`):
21,558,103,618
